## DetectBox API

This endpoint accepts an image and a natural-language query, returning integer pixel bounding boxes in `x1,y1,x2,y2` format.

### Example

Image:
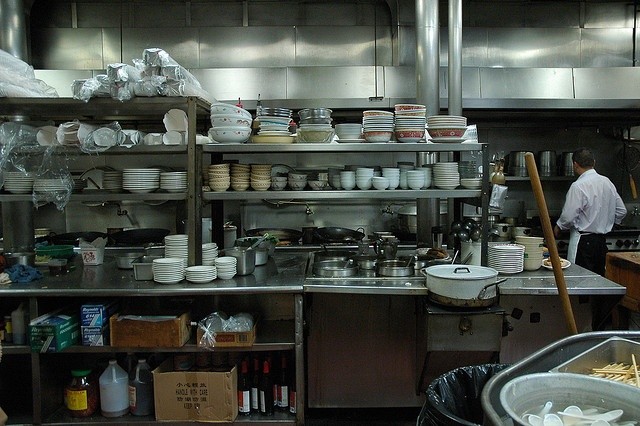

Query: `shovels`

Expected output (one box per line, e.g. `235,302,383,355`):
492,158,505,184
488,160,496,182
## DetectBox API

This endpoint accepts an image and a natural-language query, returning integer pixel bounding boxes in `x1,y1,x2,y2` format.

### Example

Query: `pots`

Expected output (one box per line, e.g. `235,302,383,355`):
4,252,36,269
242,227,306,242
312,226,365,243
48,231,109,247
110,228,170,246
224,247,255,275
419,263,508,300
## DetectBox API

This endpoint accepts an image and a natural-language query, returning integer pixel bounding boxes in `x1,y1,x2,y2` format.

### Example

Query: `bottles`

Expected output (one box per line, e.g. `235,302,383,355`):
238,360,252,416
4,315,13,343
274,356,296,415
260,359,274,416
250,359,260,414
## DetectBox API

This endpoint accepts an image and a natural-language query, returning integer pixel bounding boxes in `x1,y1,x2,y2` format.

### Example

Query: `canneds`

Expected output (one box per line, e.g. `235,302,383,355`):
3,315,14,342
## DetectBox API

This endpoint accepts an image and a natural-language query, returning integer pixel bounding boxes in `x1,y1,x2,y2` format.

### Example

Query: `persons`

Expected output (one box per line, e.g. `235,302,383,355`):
554,149,628,277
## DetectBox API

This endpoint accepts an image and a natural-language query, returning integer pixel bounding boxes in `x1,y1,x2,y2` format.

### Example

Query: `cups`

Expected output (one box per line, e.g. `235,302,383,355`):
432,226,443,249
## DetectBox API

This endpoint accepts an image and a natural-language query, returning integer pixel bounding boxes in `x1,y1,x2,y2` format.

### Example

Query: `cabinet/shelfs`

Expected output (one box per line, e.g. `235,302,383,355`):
1,96,226,268
198,143,489,269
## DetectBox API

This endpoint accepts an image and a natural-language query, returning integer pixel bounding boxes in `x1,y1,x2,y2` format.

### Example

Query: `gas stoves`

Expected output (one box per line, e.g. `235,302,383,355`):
544,225,640,237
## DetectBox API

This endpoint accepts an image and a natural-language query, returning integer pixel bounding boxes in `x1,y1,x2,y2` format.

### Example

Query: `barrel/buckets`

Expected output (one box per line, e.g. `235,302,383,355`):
100,360,128,417
131,357,154,416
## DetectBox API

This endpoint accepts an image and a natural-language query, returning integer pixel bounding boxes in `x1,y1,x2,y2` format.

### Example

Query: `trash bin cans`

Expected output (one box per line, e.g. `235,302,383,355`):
416,363,513,425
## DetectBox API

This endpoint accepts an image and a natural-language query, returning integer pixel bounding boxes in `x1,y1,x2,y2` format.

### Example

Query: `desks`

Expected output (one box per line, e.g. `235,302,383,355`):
0,252,308,426
604,252,640,331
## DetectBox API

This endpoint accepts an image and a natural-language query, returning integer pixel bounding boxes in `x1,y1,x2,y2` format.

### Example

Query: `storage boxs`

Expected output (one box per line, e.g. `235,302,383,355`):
152,356,239,424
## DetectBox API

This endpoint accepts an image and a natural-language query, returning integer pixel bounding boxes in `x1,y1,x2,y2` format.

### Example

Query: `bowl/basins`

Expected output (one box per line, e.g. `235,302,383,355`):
207,163,231,192
250,164,271,190
298,116,333,124
230,163,251,191
255,247,269,265
308,180,327,190
210,103,252,118
215,257,237,279
313,248,413,276
113,252,146,268
394,104,427,143
297,130,334,142
287,172,308,190
34,245,74,260
271,176,287,181
427,116,468,137
270,180,287,190
335,123,362,139
298,107,332,119
515,236,544,270
363,110,394,142
210,125,252,145
299,124,332,130
500,372,639,426
318,161,432,190
209,114,252,127
489,214,532,236
202,243,219,265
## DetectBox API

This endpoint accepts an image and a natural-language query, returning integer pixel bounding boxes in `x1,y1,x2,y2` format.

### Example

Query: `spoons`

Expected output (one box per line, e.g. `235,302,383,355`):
521,401,638,426
448,218,500,242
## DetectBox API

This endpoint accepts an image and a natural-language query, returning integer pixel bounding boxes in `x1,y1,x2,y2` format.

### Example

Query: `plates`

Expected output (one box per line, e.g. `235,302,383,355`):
433,162,460,190
3,170,33,194
152,258,185,285
102,171,122,191
160,171,188,194
488,243,525,274
34,178,71,194
461,178,482,188
72,179,84,193
542,258,571,270
335,139,365,143
122,168,162,193
428,139,467,143
254,107,293,135
459,161,477,178
186,265,218,283
164,234,188,277
